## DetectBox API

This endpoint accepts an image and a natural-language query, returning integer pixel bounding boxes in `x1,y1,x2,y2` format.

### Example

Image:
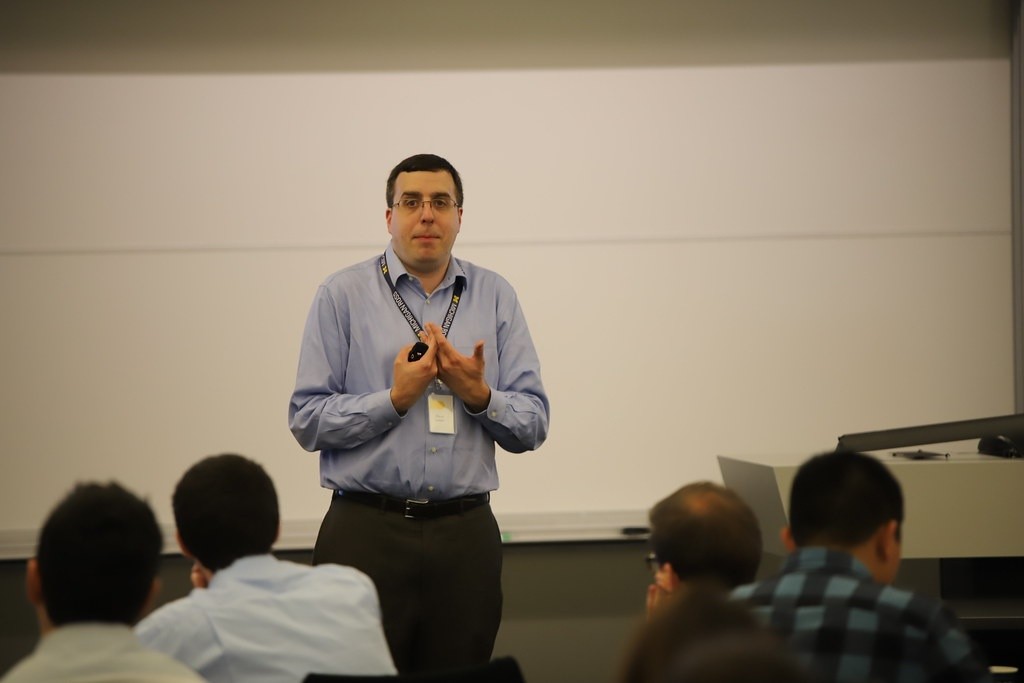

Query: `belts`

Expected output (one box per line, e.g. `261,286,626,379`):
336,489,488,517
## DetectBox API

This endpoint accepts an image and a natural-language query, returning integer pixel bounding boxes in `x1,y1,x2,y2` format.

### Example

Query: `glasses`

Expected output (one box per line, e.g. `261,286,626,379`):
391,198,460,211
643,556,665,576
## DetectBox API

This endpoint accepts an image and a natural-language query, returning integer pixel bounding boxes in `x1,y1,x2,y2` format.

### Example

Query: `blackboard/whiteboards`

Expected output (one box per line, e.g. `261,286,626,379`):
0,50,1023,562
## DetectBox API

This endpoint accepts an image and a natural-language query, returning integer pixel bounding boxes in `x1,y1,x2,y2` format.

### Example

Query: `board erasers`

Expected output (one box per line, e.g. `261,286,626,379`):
619,527,650,535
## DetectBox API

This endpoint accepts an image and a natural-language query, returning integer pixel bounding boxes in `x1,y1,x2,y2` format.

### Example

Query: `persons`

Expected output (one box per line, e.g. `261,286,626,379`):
287,153,552,683
644,482,763,621
0,480,208,683
726,451,993,683
613,589,812,683
132,454,398,683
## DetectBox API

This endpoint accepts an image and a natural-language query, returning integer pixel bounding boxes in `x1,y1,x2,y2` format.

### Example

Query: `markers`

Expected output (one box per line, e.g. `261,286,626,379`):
501,532,510,541
